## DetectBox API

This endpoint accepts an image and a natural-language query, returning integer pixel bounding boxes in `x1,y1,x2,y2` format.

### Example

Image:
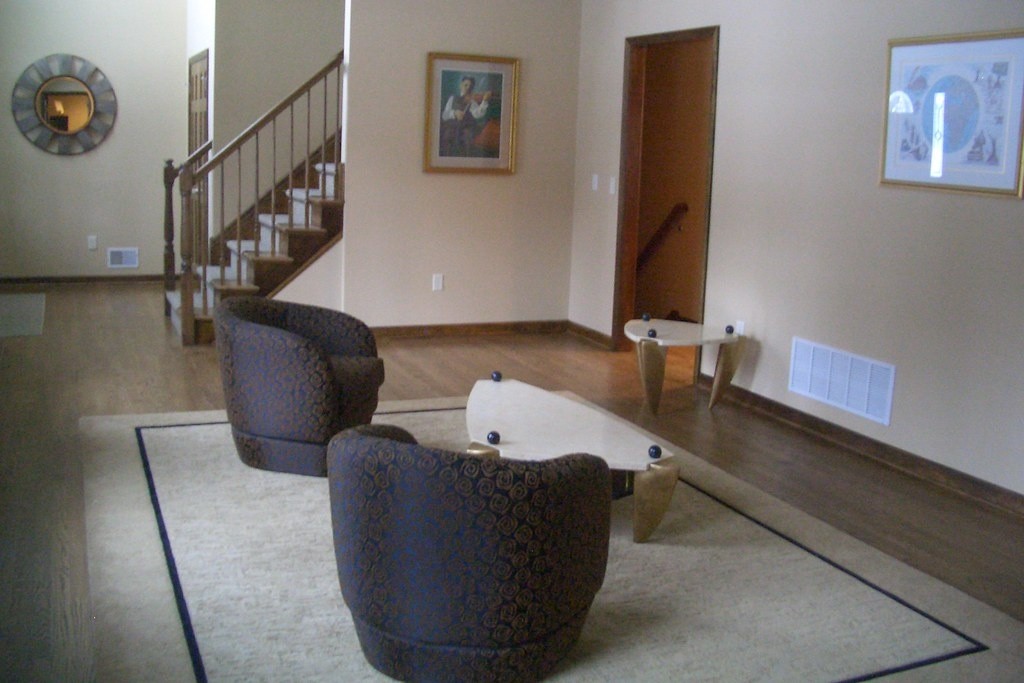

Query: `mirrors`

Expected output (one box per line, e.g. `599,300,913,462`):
12,54,117,155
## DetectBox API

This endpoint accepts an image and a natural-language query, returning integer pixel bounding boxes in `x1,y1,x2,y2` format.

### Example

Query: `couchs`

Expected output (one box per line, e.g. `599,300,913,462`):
328,425,614,683
213,295,384,479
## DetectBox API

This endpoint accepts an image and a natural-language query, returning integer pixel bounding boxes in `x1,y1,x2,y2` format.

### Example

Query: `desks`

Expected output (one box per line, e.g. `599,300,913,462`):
624,313,740,417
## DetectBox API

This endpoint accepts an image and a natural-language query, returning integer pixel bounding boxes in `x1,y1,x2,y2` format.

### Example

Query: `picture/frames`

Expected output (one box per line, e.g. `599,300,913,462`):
878,29,1024,200
423,51,522,176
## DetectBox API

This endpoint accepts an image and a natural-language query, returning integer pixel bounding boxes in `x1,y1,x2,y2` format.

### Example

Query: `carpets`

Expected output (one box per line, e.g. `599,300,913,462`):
0,292,46,338
79,390,1024,683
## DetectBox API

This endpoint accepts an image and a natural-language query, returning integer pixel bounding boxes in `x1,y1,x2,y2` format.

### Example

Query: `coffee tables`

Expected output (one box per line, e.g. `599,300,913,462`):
466,371,679,544
